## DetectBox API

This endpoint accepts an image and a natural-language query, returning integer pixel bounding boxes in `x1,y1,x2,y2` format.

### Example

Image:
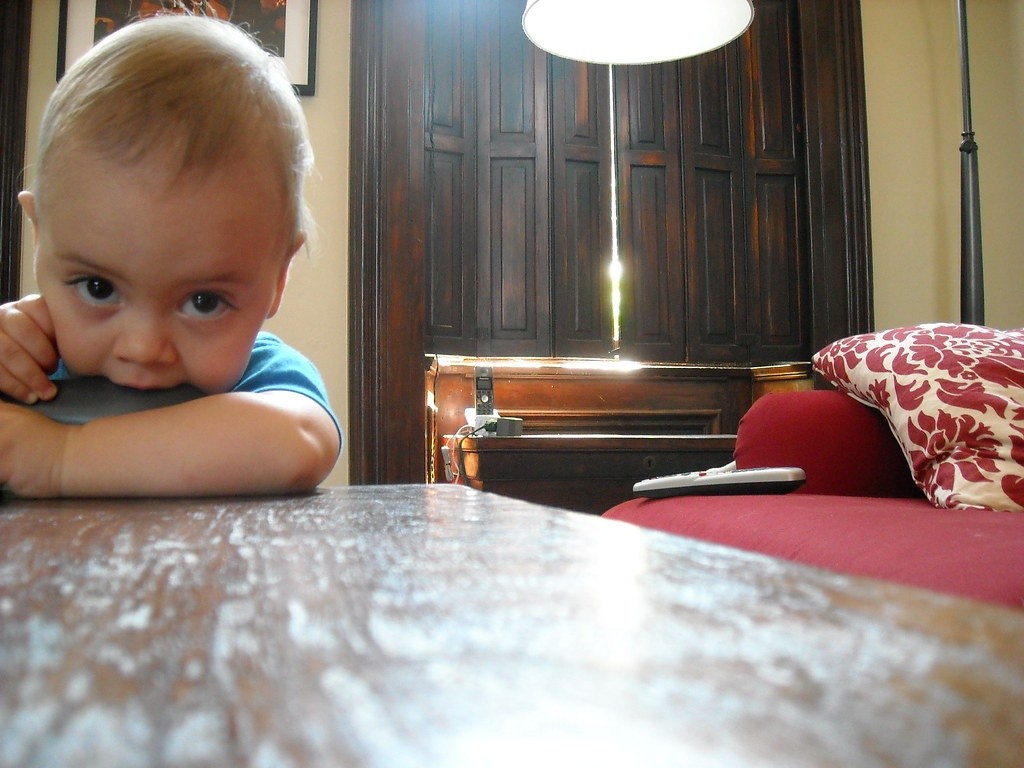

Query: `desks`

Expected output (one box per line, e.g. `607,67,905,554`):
0,482,1024,768
439,434,737,515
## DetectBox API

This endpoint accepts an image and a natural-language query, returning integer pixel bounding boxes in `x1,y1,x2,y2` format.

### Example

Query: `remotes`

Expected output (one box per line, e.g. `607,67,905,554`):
633,466,807,498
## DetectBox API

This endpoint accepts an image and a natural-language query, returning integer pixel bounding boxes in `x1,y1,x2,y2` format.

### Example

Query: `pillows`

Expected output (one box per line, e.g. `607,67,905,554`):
813,320,1024,514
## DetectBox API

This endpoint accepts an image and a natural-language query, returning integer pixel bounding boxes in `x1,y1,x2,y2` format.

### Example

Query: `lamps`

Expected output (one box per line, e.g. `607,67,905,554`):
522,0,754,65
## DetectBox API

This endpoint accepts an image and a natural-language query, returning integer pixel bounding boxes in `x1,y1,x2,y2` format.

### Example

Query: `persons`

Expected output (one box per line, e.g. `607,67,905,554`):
0,14,343,499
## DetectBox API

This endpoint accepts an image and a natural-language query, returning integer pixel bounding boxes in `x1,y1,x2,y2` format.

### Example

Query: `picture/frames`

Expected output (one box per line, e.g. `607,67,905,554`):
54,0,320,98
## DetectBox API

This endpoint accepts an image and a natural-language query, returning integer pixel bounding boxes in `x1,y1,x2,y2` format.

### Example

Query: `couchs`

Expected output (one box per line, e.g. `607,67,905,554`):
606,388,1024,625
